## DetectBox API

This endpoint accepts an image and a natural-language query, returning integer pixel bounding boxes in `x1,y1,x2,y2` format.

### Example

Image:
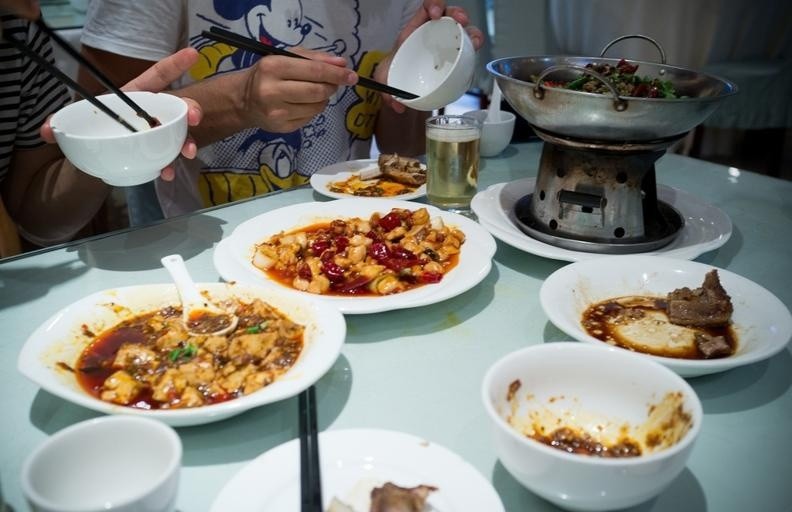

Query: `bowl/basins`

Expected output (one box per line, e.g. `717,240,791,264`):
462,109,516,158
482,343,704,511
20,417,184,511
49,91,189,188
387,16,478,113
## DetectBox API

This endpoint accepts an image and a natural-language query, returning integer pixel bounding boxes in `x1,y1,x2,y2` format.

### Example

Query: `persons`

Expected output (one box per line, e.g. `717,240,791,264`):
79,0,485,228
0,1,204,258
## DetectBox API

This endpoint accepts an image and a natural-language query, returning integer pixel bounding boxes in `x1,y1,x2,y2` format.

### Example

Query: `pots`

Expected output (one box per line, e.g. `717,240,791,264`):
487,35,740,146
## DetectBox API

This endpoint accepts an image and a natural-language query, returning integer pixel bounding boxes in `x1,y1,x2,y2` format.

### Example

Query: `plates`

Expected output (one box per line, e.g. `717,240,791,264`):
540,257,792,380
207,428,506,511
309,159,426,200
213,198,497,316
17,283,347,427
470,175,732,262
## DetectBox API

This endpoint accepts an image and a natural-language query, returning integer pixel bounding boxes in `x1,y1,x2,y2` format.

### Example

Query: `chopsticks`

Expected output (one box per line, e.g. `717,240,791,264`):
298,385,324,512
202,26,420,99
1,1,159,134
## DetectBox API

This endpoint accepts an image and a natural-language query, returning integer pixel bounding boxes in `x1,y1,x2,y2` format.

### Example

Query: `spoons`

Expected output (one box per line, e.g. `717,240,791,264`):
161,253,239,337
485,78,502,124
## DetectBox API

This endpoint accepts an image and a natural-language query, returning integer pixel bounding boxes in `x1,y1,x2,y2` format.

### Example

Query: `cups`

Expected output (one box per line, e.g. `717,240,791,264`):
424,116,481,211
